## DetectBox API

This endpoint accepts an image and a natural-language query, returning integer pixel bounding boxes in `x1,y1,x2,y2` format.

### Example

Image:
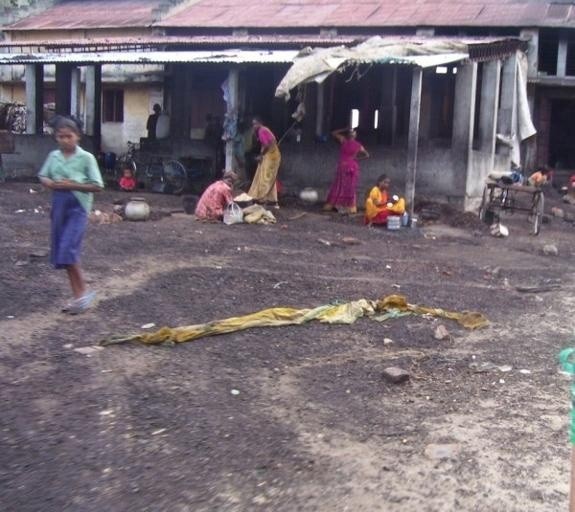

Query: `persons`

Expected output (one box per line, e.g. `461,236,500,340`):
38,116,107,315
527,167,552,188
233,112,260,185
365,173,406,227
147,102,164,139
319,126,372,215
118,168,136,192
190,170,239,223
246,115,283,209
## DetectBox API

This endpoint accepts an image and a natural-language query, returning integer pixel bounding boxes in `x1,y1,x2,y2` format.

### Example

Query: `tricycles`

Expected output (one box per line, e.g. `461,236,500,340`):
478,176,546,236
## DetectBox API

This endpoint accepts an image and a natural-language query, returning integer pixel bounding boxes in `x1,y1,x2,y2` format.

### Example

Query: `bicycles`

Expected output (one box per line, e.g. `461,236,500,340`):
116,141,188,195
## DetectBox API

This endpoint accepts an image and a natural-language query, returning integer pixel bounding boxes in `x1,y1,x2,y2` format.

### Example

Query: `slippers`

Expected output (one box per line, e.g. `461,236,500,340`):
61,289,97,315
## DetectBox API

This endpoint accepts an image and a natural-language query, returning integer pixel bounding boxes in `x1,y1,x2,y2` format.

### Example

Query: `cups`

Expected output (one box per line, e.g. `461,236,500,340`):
386,202,393,208
411,215,418,228
402,214,409,226
393,195,399,201
